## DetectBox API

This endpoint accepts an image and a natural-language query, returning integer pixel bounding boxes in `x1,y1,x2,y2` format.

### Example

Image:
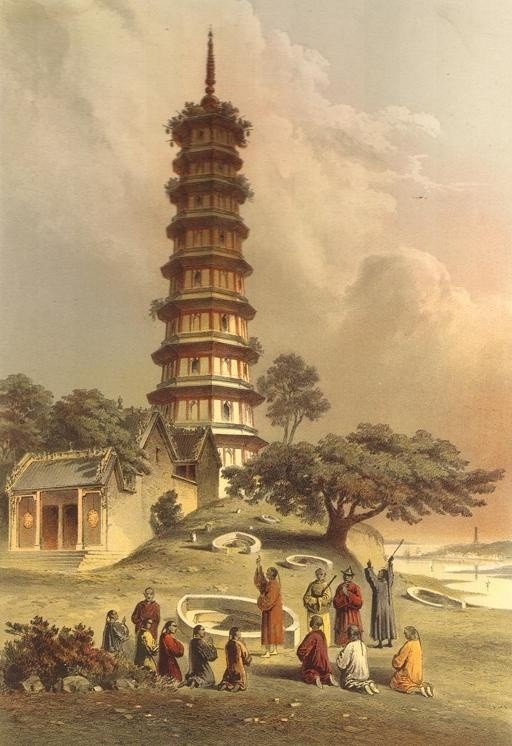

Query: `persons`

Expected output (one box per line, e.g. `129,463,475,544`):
133,617,159,674
217,626,252,692
131,587,161,640
332,566,364,646
178,624,217,689
389,626,433,698
364,555,397,648
252,554,284,657
156,620,184,681
334,624,380,696
296,615,339,689
302,567,332,648
101,609,130,660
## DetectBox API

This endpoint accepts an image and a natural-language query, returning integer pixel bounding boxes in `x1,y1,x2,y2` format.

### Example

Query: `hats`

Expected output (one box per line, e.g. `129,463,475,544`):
341,564,355,576
